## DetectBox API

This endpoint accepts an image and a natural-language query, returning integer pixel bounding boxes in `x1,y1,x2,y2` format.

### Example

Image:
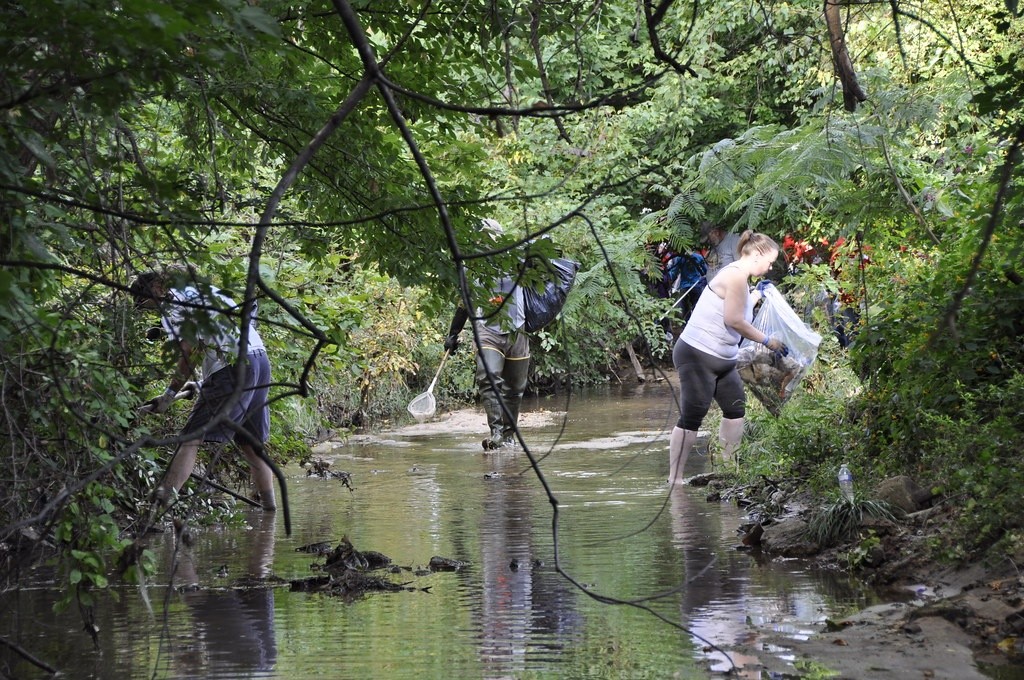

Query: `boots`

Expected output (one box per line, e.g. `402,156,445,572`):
502,354,530,446
476,347,505,449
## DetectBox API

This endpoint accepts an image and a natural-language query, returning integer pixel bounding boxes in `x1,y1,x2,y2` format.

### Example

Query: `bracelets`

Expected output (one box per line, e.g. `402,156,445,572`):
166,388,175,397
763,336,769,345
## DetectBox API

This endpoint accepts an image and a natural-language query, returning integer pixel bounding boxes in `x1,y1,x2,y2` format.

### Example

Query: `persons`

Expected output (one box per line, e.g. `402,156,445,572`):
669,246,705,325
795,255,873,346
669,222,789,484
444,219,530,449
131,262,276,510
639,242,680,336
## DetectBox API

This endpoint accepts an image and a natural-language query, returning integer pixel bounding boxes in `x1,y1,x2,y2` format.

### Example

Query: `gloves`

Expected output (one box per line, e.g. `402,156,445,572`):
752,279,772,300
763,337,789,359
444,306,469,356
144,388,175,415
182,380,205,400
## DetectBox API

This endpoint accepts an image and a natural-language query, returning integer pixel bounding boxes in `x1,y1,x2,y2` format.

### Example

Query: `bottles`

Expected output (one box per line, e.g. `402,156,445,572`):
838,464,854,502
